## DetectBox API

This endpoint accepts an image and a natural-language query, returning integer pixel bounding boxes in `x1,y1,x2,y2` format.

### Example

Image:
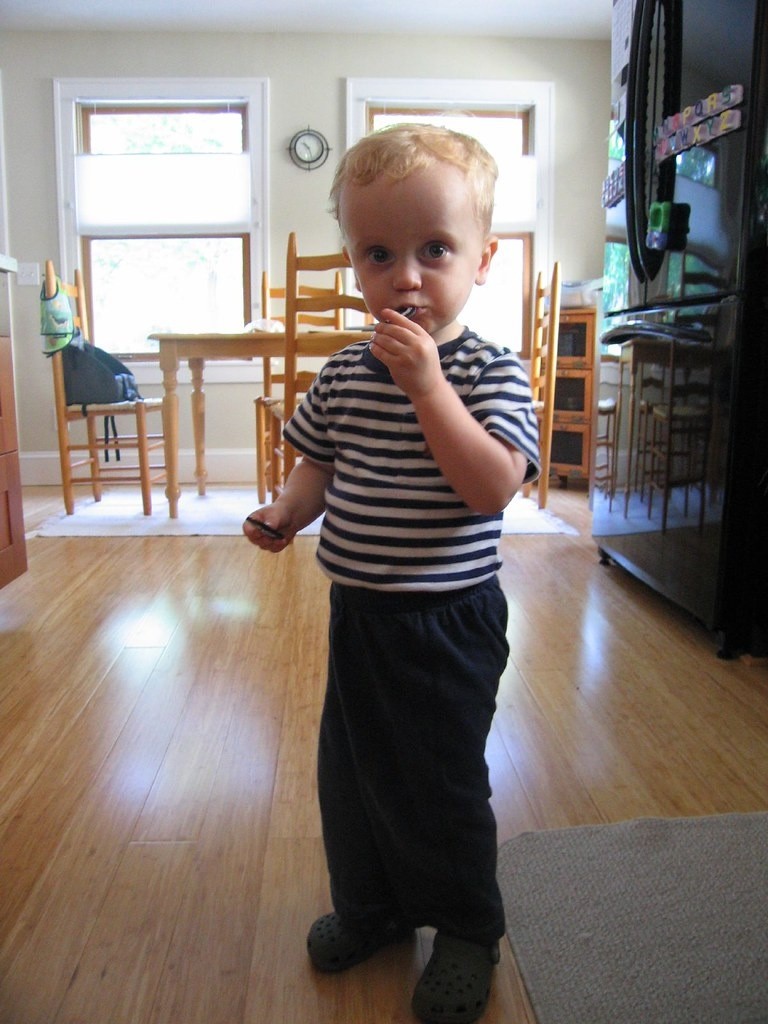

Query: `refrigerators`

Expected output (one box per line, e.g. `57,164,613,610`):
592,1,768,661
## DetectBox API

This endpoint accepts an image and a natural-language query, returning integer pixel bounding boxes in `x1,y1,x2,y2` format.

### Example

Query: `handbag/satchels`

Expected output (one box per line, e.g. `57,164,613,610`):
63,332,144,406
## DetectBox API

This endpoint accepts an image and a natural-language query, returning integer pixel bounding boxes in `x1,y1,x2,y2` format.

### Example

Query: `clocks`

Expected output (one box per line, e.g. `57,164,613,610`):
285,125,333,173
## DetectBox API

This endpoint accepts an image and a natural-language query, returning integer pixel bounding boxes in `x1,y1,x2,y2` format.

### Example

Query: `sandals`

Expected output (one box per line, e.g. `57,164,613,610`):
411,933,494,1024
307,912,415,975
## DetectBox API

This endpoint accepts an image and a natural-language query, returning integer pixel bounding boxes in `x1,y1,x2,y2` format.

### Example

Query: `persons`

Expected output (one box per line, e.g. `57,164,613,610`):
245,124,541,1023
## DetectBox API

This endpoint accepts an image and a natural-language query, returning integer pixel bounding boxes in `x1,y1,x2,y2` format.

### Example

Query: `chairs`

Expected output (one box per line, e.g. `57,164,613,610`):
634,335,674,503
39,259,163,516
595,355,623,499
253,270,343,504
268,232,374,544
647,336,715,535
522,260,561,510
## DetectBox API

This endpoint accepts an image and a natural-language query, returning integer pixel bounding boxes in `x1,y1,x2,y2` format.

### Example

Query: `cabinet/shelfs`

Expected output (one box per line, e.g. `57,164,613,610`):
532,306,598,488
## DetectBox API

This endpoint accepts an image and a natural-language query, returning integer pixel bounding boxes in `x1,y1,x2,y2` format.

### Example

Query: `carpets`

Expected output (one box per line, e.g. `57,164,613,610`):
495,811,768,1024
24,487,581,540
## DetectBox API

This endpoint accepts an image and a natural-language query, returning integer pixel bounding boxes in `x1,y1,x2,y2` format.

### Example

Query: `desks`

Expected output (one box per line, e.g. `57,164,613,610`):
609,339,711,520
147,330,374,519
